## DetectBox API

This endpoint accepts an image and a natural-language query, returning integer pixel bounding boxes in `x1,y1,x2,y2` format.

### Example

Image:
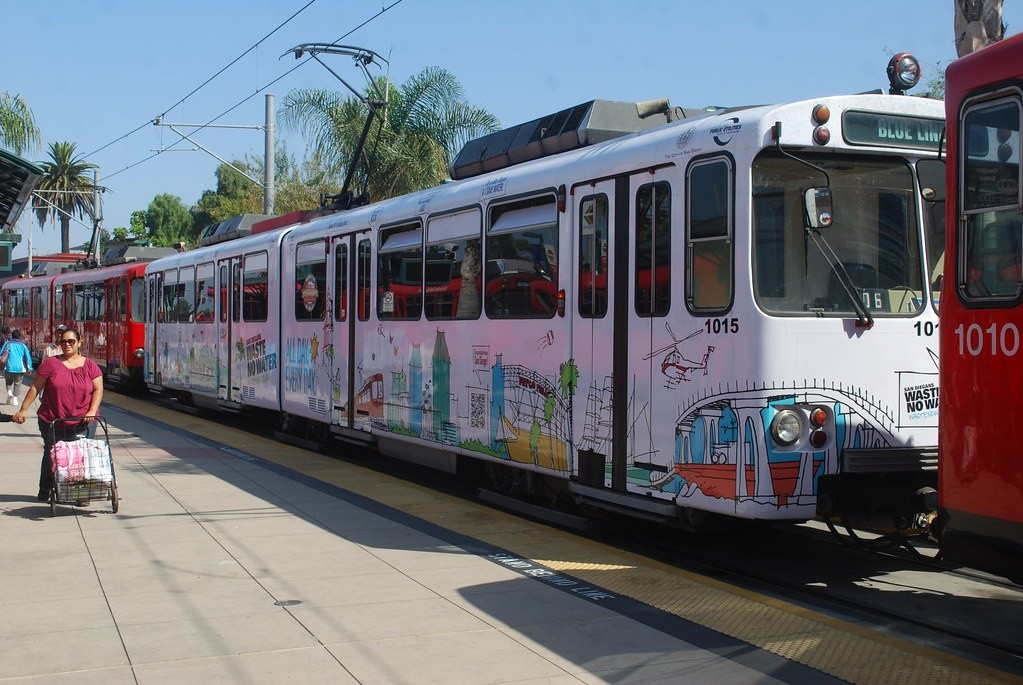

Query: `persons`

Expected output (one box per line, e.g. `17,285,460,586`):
0,325,12,376
12,329,103,507
0,330,34,406
39,325,68,400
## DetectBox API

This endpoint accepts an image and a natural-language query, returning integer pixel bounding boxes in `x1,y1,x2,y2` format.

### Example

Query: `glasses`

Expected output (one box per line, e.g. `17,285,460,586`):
60,338,80,345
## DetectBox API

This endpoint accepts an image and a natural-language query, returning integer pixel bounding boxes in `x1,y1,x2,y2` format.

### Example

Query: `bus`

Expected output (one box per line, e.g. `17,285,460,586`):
0,246,178,383
940,30,1023,549
145,88,1023,541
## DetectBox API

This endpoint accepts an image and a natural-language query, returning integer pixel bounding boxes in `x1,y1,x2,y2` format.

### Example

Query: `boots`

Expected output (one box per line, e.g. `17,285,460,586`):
6,390,14,404
13,397,18,405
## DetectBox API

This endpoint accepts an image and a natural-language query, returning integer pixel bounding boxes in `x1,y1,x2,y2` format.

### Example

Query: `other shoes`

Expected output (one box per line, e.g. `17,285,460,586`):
77,500,90,506
38,488,51,500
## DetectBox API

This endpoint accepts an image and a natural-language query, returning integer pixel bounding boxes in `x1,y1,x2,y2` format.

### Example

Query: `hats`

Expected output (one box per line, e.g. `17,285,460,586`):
55,324,67,332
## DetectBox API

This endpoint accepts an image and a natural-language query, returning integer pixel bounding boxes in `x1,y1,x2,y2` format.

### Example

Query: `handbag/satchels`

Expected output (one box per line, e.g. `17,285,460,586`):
0,340,10,371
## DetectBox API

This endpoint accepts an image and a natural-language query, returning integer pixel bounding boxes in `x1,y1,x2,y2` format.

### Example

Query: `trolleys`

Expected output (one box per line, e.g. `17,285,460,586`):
46,415,122,516
